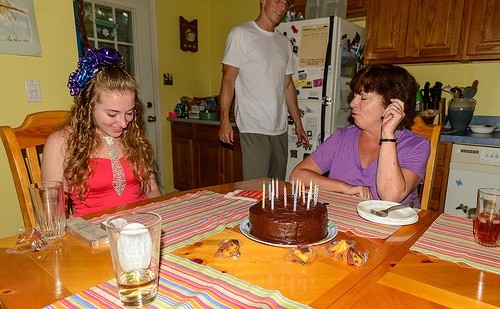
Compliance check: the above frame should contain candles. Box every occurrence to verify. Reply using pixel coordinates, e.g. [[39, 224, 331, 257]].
[[262, 177, 318, 211]]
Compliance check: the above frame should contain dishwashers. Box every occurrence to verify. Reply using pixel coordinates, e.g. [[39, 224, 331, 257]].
[[443, 144, 500, 219]]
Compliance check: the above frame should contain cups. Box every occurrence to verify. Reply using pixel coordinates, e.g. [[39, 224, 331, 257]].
[[28, 181, 67, 240], [104, 212, 162, 305], [420, 111, 437, 125], [474, 188, 500, 247]]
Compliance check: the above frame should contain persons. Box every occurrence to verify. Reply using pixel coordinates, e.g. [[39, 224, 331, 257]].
[[217, 0, 312, 181], [289, 61, 432, 211], [40, 44, 164, 225]]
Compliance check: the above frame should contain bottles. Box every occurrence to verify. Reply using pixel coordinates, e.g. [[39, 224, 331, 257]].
[[183, 98, 189, 119], [448, 97, 477, 131], [414, 85, 422, 115]]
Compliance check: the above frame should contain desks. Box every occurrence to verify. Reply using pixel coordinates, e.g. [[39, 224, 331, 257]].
[[0, 177, 500, 309]]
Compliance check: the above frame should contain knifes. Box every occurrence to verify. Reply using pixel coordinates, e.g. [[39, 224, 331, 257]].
[[422, 81, 444, 111]]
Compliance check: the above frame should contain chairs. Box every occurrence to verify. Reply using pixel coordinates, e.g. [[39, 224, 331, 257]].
[[410, 124, 443, 210], [0, 111, 74, 230]]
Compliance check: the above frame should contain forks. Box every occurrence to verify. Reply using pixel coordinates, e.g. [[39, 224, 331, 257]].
[[370, 199, 418, 217]]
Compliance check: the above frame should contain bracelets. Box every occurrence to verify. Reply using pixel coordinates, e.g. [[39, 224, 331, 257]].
[[378, 138, 399, 147]]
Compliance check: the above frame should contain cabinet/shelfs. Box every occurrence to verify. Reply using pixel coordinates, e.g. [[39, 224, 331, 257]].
[[430, 141, 453, 212], [170, 120, 242, 190], [289, 0, 500, 64]]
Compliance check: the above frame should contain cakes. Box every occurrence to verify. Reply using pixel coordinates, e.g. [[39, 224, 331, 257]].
[[248, 193, 329, 245]]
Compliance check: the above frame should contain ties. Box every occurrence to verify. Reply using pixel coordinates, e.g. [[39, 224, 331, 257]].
[[101, 136, 126, 196]]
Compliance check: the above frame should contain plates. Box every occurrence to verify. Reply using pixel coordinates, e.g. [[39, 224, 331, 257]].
[[238, 216, 339, 248], [356, 199, 418, 225], [469, 124, 498, 134]]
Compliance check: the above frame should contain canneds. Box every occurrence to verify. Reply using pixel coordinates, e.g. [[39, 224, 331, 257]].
[[176, 103, 184, 118]]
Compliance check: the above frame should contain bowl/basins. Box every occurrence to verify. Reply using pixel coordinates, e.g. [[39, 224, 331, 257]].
[[199, 110, 218, 121]]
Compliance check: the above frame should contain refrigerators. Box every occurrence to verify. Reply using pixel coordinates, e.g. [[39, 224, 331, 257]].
[[276, 16, 367, 180]]
